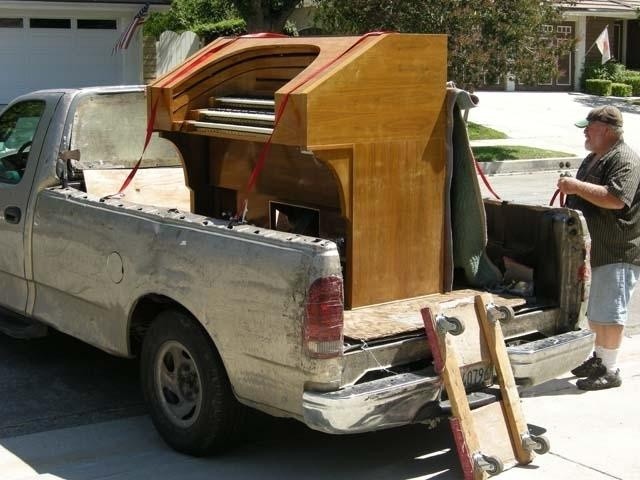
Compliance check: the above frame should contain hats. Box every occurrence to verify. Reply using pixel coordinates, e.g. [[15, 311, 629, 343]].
[[573, 105, 623, 129]]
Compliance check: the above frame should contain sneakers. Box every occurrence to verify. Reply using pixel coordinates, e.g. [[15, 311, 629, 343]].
[[571, 351, 622, 391]]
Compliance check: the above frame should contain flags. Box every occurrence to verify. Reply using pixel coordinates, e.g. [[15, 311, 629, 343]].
[[596, 27, 611, 65]]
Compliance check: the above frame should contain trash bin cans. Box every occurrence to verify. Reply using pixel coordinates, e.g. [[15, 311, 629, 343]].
[[503, 74, 516, 92]]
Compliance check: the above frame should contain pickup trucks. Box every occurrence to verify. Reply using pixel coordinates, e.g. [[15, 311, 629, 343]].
[[0, 83, 599, 456]]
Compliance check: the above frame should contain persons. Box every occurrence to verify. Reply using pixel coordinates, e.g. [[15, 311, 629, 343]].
[[557, 107, 639, 391]]
